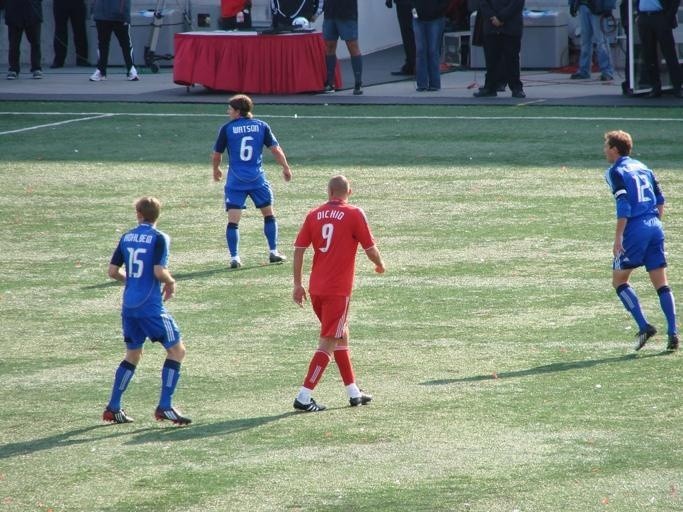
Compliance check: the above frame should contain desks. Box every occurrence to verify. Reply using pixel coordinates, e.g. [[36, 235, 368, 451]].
[[174, 26, 341, 96]]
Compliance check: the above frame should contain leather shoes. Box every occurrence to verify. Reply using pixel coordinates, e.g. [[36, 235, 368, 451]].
[[473, 85, 506, 97], [644, 89, 661, 98], [391, 67, 442, 91], [511, 90, 526, 97]]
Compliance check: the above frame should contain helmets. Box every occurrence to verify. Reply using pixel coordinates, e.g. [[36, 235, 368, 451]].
[[291, 16, 310, 31]]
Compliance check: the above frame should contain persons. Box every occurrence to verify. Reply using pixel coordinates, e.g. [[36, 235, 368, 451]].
[[210, 94, 291, 269], [385, 1, 527, 98], [100, 195, 191, 426], [219, 0, 365, 95], [2, 1, 140, 82], [289, 175, 385, 411], [567, 1, 683, 99], [599, 129, 681, 352]]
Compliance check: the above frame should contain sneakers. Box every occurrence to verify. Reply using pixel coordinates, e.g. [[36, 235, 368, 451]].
[[230, 257, 243, 270], [600, 73, 614, 82], [32, 63, 64, 79], [103, 406, 136, 424], [5, 70, 20, 81], [293, 398, 327, 412], [127, 65, 139, 80], [665, 333, 679, 352], [75, 61, 107, 81], [269, 252, 289, 263], [320, 84, 337, 94], [155, 406, 191, 427], [572, 69, 591, 79], [352, 84, 364, 94], [634, 323, 657, 351], [349, 391, 373, 406]]
